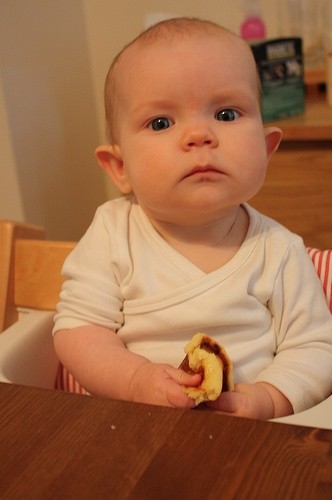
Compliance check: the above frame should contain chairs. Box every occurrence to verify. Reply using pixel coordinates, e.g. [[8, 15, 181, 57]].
[[0, 218, 332, 426]]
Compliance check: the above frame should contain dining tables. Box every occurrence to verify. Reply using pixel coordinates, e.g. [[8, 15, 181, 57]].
[[0, 380, 332, 500]]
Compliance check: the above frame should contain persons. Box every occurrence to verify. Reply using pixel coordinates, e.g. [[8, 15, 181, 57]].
[[52, 18, 331, 429]]
